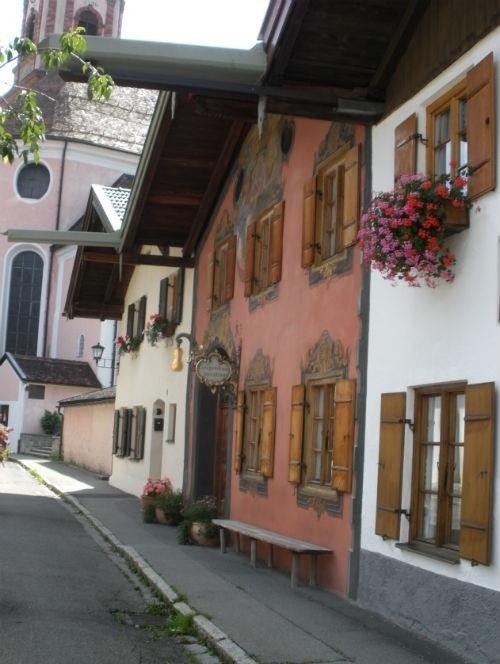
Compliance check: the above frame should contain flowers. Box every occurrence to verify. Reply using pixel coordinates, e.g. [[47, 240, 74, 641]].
[[143, 478, 173, 496], [176, 496, 230, 545], [142, 314, 172, 347], [116, 335, 143, 360], [356, 160, 480, 289]]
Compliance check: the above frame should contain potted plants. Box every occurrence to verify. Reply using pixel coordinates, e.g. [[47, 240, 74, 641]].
[[143, 489, 183, 526]]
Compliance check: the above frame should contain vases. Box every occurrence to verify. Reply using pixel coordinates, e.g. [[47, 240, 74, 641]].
[[141, 495, 154, 511], [162, 323, 174, 336], [187, 522, 220, 545], [442, 205, 469, 240]]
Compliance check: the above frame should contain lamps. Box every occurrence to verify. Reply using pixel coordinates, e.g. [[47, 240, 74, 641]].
[[92, 342, 120, 375], [172, 333, 199, 371]]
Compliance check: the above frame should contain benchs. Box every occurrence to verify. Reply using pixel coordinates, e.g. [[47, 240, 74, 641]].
[[212, 519, 332, 588]]
